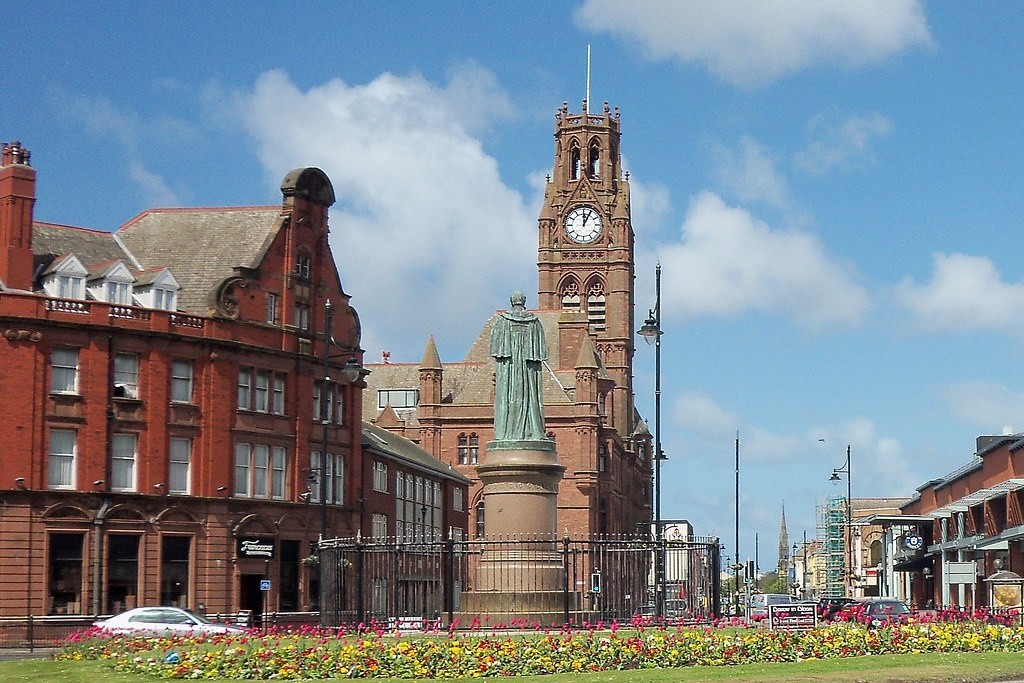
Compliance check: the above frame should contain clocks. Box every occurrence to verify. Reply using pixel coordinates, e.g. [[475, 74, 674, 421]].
[[564, 206, 603, 244]]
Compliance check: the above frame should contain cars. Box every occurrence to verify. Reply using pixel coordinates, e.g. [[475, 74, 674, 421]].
[[633, 582, 880, 625], [857, 599, 913, 632], [93, 606, 245, 641]]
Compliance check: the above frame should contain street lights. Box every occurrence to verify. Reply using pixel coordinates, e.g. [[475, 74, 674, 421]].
[[635, 258, 669, 628], [792, 530, 807, 600], [829, 444, 852, 597]]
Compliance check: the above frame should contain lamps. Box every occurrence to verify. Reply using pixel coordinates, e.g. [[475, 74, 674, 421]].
[[923, 567, 934, 579], [93, 480, 105, 493], [154, 482, 166, 495], [14, 478, 27, 490], [299, 491, 313, 504], [993, 559, 1008, 573], [217, 485, 228, 497]]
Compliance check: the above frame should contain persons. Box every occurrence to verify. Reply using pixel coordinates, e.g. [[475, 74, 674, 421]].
[[489, 290, 548, 441]]
